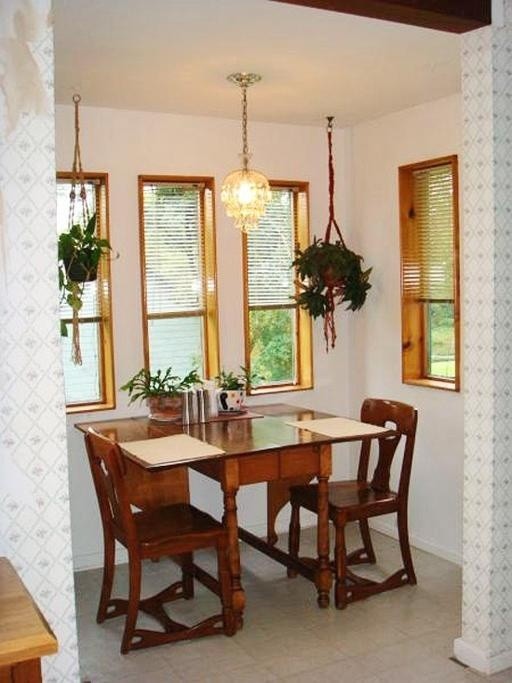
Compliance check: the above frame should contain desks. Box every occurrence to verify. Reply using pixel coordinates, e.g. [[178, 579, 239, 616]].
[[74, 405, 400, 631]]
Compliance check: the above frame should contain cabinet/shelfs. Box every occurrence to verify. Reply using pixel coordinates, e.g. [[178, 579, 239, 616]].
[[1, 556, 58, 683]]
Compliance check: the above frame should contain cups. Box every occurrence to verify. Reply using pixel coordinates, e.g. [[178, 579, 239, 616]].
[[198, 388, 210, 422], [182, 390, 198, 425]]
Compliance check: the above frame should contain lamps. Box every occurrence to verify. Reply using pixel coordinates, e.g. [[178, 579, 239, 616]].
[[221, 72, 270, 231]]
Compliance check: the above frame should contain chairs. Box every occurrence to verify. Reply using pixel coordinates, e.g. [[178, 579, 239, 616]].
[[286, 397, 419, 611], [84, 428, 236, 653]]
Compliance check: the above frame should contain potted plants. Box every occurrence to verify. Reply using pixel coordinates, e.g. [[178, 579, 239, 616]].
[[120, 368, 204, 421], [58, 211, 111, 337], [215, 366, 267, 413], [290, 238, 371, 320]]
